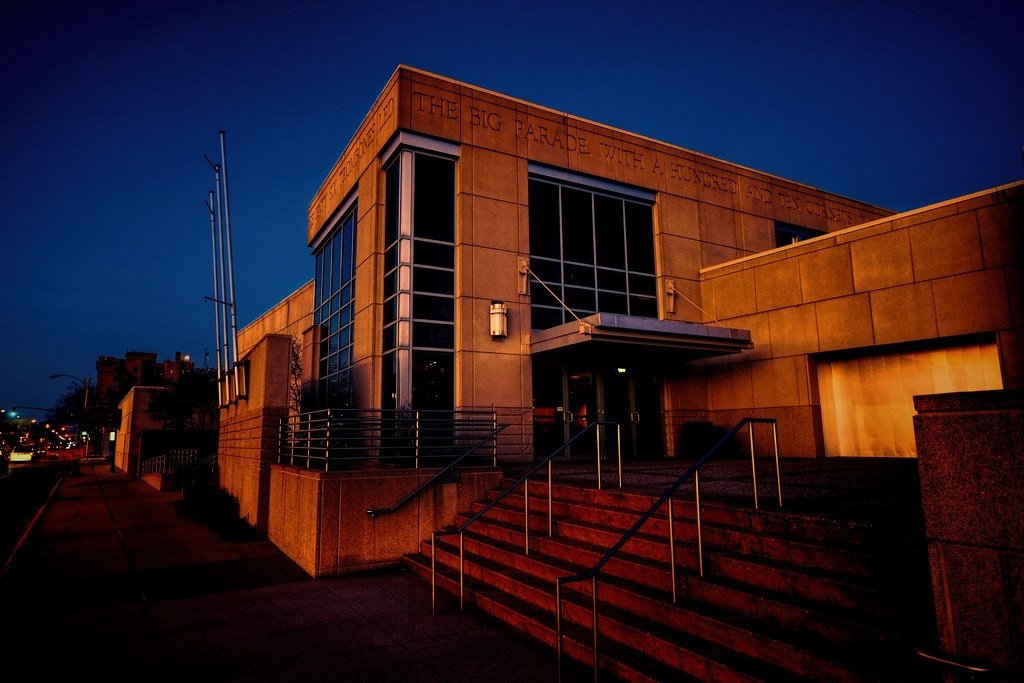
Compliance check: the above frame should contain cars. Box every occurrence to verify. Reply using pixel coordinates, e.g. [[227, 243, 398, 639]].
[[0, 449, 10, 475], [4, 442, 64, 462]]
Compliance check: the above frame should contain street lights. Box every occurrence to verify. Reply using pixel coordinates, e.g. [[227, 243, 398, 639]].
[[49, 373, 90, 458]]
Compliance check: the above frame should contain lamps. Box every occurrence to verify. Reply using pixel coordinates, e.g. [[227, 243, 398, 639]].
[[489, 303, 507, 336]]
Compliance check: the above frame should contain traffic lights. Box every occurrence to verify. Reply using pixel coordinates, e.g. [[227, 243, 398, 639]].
[[11, 407, 16, 417]]
